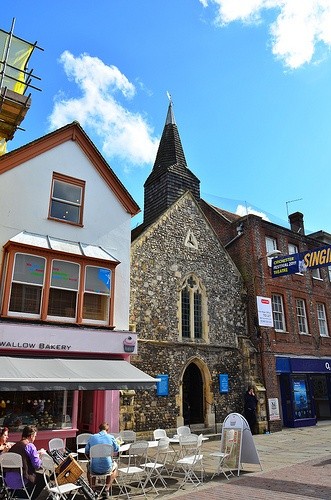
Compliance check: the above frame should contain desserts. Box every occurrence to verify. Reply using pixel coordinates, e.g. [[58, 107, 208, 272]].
[[123, 336, 137, 352], [30, 411, 53, 427]]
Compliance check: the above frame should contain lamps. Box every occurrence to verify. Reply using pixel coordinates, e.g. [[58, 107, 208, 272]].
[[258, 249, 283, 262]]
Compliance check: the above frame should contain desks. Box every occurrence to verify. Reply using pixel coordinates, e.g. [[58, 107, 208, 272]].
[[132, 437, 169, 463], [168, 434, 209, 457]]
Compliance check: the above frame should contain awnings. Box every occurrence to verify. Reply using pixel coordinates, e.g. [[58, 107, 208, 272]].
[[0, 356, 162, 392]]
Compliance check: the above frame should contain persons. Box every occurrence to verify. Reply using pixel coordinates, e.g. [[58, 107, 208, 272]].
[[0, 427, 13, 485], [244, 387, 258, 436], [85, 423, 119, 496], [4, 426, 50, 500]]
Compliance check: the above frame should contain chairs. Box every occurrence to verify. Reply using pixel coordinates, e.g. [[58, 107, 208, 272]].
[[39, 452, 83, 500], [0, 452, 38, 500], [209, 429, 240, 482], [116, 440, 150, 500], [121, 429, 138, 467], [175, 432, 204, 491], [176, 426, 195, 456], [48, 437, 65, 455], [140, 435, 171, 492], [87, 443, 115, 499], [153, 429, 178, 469], [75, 433, 94, 465]]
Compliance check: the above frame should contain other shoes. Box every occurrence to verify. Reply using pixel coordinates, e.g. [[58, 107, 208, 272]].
[[105, 487, 109, 497]]
